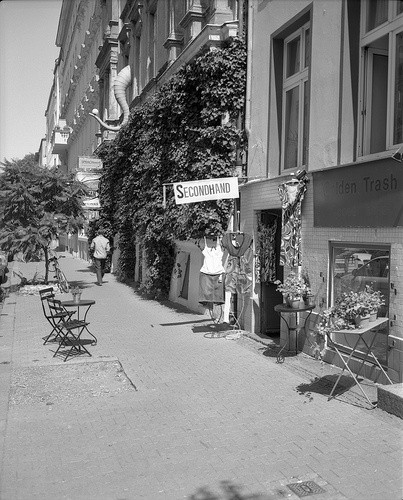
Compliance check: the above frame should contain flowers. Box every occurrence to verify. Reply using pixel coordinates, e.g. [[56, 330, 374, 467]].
[[310, 285, 386, 359], [275, 271, 312, 299]]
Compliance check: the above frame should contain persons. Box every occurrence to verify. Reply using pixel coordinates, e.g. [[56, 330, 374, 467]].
[[91, 229, 111, 285]]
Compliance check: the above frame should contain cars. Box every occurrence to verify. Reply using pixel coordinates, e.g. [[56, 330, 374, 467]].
[[341, 255, 390, 316]]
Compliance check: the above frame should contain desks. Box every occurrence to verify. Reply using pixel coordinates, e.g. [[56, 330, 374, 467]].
[[62, 299, 98, 346], [325, 317, 393, 408], [274, 302, 316, 364]]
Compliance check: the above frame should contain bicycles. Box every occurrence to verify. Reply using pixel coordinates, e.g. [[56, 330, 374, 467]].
[[46, 255, 72, 293]]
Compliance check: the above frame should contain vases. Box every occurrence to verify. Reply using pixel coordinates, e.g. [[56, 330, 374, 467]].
[[356, 309, 379, 329], [287, 296, 300, 309]]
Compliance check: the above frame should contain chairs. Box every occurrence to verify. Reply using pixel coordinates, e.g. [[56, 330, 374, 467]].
[[38, 287, 91, 360]]
